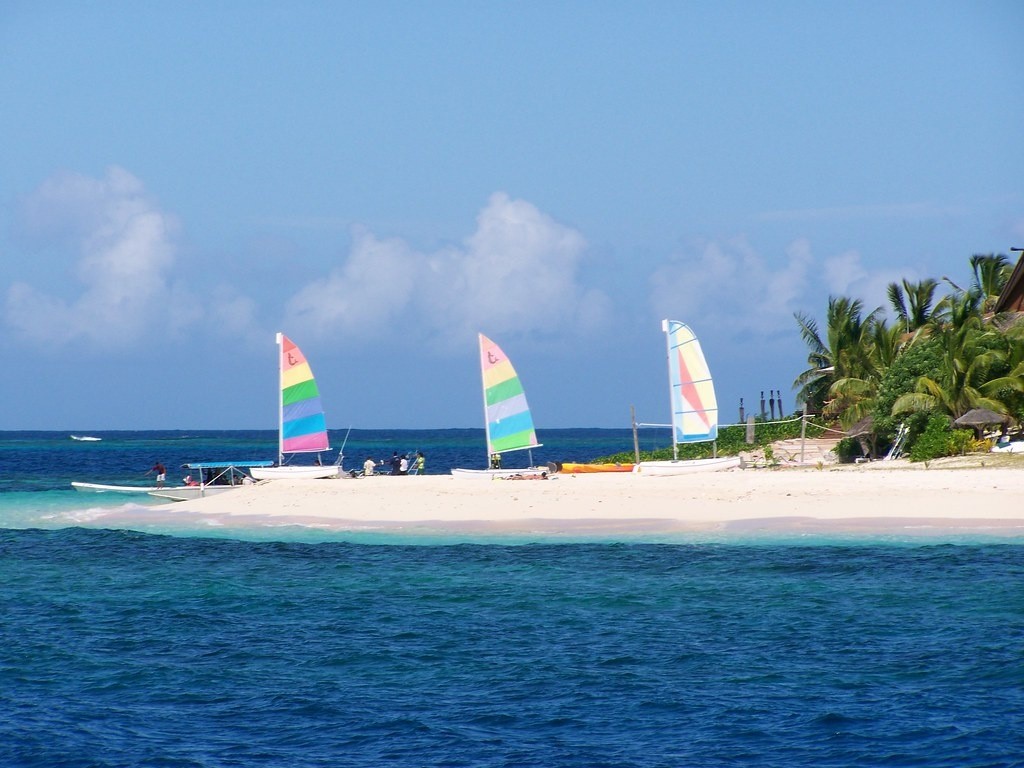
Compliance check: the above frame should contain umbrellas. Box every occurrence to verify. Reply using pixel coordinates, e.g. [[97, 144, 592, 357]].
[[847, 416, 879, 460], [955, 407, 1005, 441]]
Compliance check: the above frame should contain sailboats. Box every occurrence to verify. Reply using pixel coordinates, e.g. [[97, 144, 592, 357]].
[[249, 332, 339, 479], [631, 319, 741, 476], [450, 333, 547, 478]]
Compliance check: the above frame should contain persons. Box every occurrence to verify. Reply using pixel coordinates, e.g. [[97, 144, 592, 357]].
[[412, 452, 425, 475], [204, 468, 245, 485], [144, 461, 166, 489], [315, 460, 320, 466], [387, 451, 408, 475], [363, 456, 382, 476]]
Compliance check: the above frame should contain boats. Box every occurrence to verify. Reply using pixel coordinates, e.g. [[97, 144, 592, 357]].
[[70, 435, 102, 442], [71, 482, 171, 495], [147, 461, 275, 502], [562, 462, 636, 473]]
